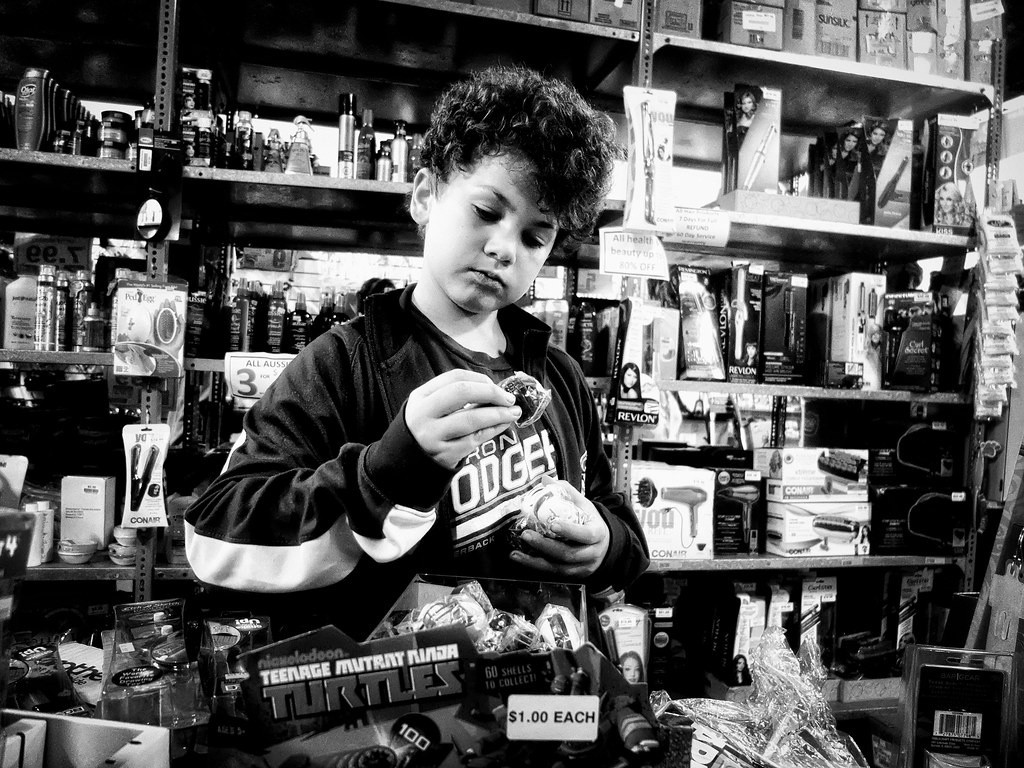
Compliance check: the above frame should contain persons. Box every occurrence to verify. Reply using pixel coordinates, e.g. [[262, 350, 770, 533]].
[[620, 651, 645, 683], [182, 66, 650, 608], [866, 121, 893, 178], [828, 139, 839, 180], [839, 129, 864, 187], [736, 92, 757, 150], [729, 654, 753, 686], [619, 362, 644, 400], [148, 484, 160, 497], [934, 182, 971, 227]]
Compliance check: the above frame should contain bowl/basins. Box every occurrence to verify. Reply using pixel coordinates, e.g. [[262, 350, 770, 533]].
[[59, 539, 99, 553], [57, 550, 94, 564]]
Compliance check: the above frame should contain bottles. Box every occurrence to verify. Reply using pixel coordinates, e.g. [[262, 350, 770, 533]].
[[1, 67, 427, 183], [0, 261, 358, 359], [35, 500, 54, 563], [23, 503, 42, 568]]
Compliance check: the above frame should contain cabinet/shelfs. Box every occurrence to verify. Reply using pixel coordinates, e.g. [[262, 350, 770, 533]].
[[3, 1, 1024, 768]]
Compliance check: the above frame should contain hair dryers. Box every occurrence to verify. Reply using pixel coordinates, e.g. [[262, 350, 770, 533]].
[[625, 454, 769, 560]]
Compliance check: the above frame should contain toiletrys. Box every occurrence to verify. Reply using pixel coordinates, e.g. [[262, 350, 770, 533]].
[[2, 51, 607, 383]]
